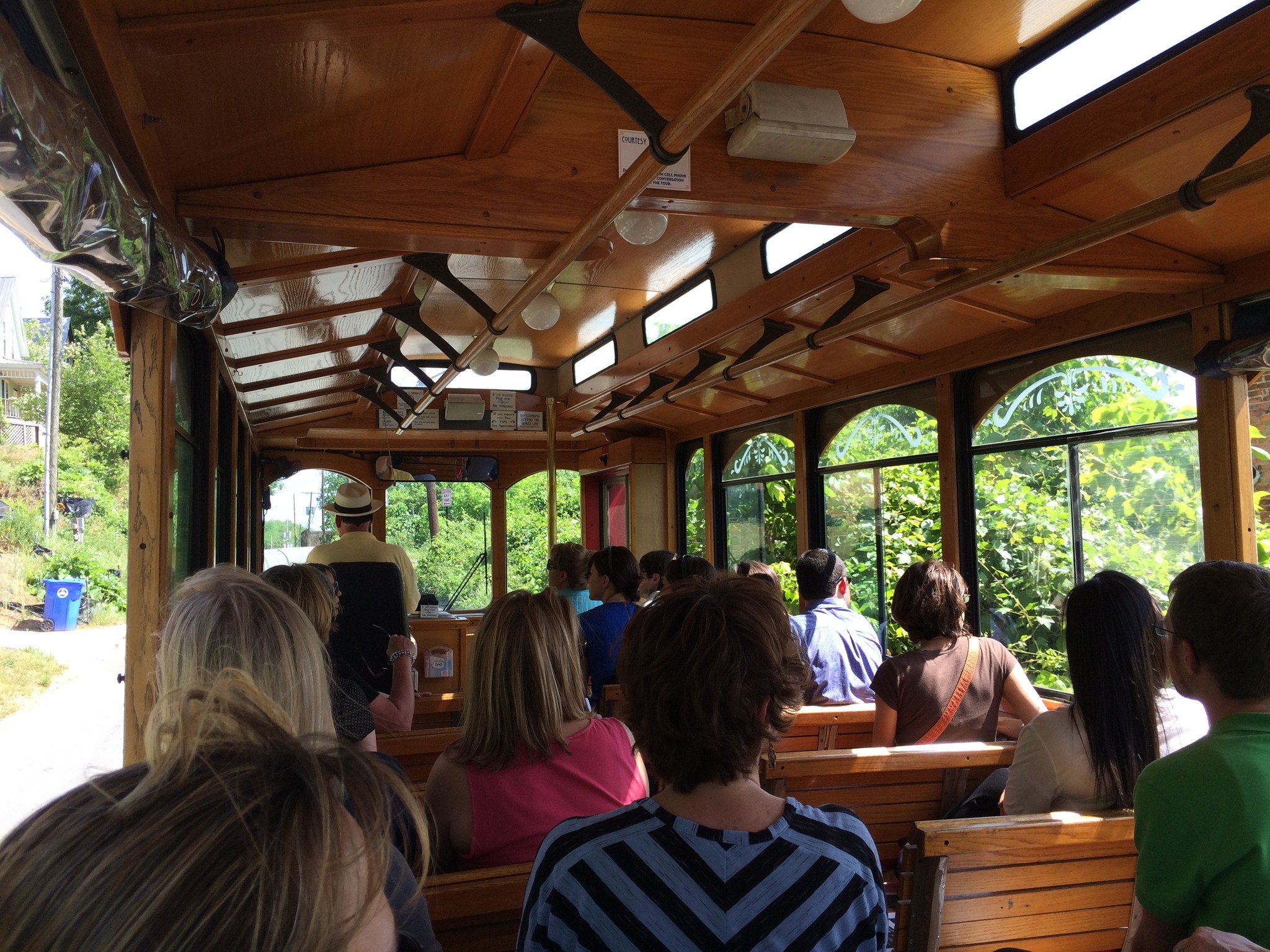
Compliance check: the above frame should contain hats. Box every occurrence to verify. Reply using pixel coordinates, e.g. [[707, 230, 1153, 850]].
[[320, 484, 387, 518]]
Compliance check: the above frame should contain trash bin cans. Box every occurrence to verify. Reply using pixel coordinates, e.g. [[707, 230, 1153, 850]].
[[40, 578, 86, 632]]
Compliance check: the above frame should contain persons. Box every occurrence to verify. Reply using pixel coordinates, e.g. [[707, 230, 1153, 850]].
[[868, 557, 1049, 746], [882, 570, 1210, 895], [146, 565, 450, 951], [425, 587, 648, 869], [783, 548, 893, 704], [544, 542, 792, 711], [304, 483, 421, 660], [301, 563, 433, 734], [0, 670, 431, 951], [514, 570, 890, 952], [255, 565, 377, 752], [376, 456, 498, 481], [1120, 557, 1270, 952]]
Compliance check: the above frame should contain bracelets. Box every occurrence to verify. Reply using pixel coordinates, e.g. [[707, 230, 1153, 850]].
[[390, 649, 416, 666]]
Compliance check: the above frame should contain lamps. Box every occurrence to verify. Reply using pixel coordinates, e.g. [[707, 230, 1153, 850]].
[[520, 281, 561, 331], [613, 210, 668, 245], [470, 335, 500, 377]]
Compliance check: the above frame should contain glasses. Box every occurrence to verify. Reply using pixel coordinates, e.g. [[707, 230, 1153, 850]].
[[1146, 620, 1204, 670], [546, 558, 564, 571], [680, 555, 705, 569]]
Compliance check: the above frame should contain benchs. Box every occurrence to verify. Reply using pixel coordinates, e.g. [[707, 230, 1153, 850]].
[[891, 803, 1139, 952], [412, 862, 537, 952], [757, 739, 1018, 910], [770, 700, 877, 755], [376, 726, 468, 800]]
